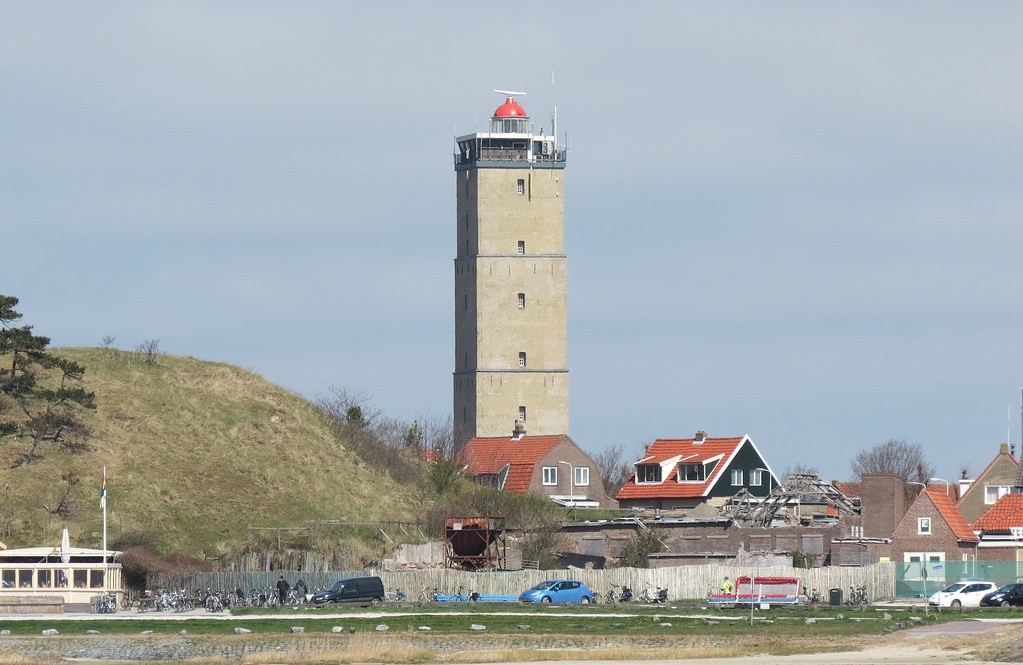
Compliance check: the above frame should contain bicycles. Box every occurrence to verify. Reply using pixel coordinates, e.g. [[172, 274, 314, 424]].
[[97, 584, 306, 615], [383, 585, 407, 602], [797, 584, 827, 605], [590, 582, 672, 605], [415, 586, 446, 603], [448, 585, 482, 601], [845, 581, 870, 605]]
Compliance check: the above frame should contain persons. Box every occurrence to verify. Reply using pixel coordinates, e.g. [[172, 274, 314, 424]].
[[10, 581, 14, 587], [22, 581, 30, 587], [293, 580, 308, 604], [396, 589, 405, 602], [720, 577, 733, 595], [277, 576, 290, 605]]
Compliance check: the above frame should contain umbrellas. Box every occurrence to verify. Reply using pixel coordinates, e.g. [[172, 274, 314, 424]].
[[60, 528, 70, 585]]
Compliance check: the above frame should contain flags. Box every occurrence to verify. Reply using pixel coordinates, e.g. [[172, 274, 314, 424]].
[[100, 477, 106, 511]]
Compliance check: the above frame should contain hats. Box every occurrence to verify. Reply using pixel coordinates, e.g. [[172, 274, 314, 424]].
[[279, 576, 283, 579]]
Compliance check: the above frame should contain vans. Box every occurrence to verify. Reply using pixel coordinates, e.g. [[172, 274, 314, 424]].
[[310, 575, 386, 610]]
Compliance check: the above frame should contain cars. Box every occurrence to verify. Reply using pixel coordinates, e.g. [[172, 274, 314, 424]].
[[980, 581, 1023, 608], [928, 580, 999, 608], [518, 579, 593, 605]]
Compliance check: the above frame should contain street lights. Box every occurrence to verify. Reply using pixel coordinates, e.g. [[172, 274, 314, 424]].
[[929, 477, 949, 495], [755, 467, 771, 495], [557, 460, 573, 503]]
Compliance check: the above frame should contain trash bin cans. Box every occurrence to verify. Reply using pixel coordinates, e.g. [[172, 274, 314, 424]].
[[829, 588, 844, 606]]
